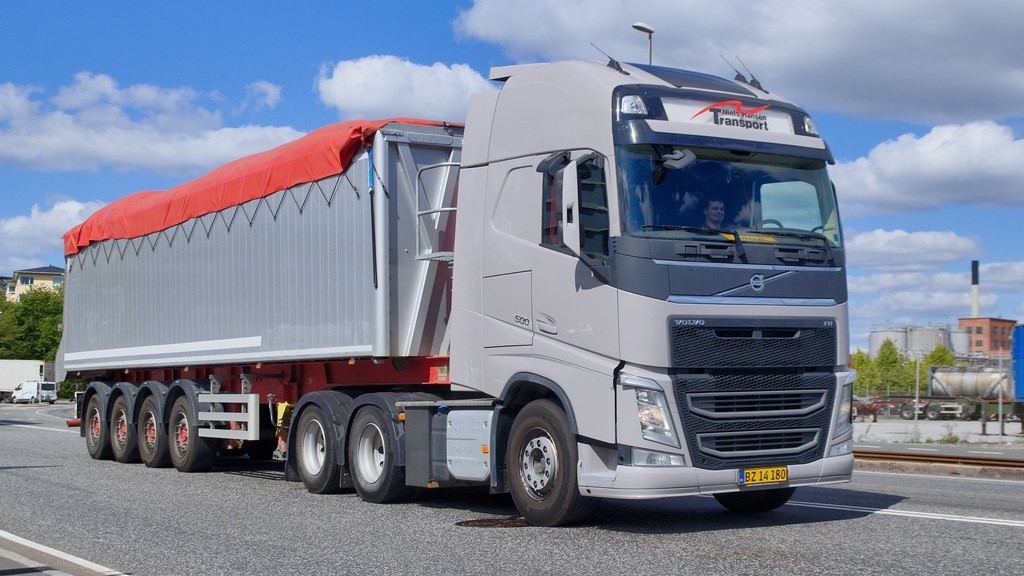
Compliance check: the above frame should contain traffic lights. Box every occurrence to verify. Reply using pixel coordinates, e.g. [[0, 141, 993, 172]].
[[39, 365, 44, 376]]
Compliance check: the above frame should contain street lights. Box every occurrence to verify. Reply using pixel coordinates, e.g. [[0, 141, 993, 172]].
[[632, 20, 657, 65]]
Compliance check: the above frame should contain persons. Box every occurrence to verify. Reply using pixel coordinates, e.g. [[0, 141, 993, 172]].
[[692, 197, 728, 228]]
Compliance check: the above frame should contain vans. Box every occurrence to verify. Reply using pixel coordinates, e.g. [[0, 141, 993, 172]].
[[11, 380, 57, 405]]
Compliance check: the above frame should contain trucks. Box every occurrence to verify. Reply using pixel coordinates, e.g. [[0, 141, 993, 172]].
[[0, 358, 45, 403]]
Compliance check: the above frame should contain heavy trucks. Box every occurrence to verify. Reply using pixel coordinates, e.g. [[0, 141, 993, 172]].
[[54, 58, 861, 527], [851, 322, 1024, 434]]
